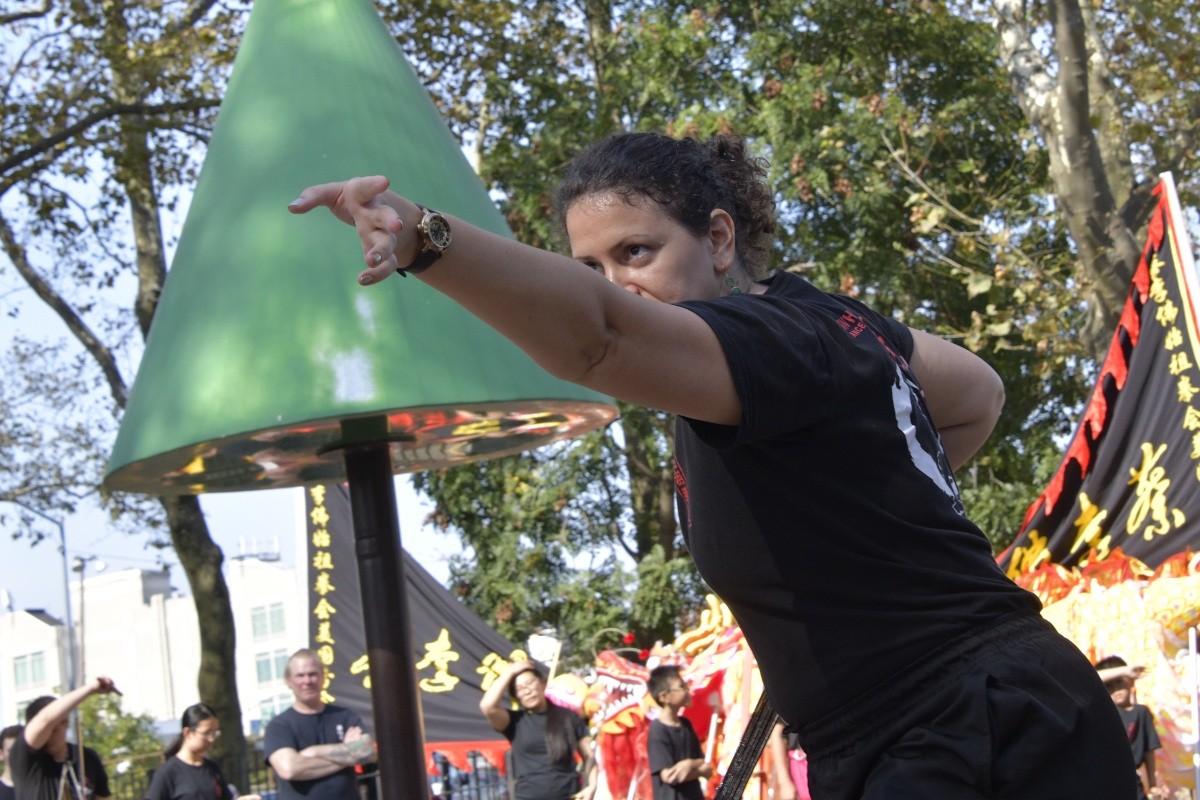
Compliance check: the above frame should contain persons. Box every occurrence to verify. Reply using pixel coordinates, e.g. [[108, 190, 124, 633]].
[[264, 648, 379, 799], [9, 674, 114, 800], [760, 723, 811, 800], [479, 659, 599, 800], [0, 724, 26, 800], [1092, 654, 1163, 800], [287, 131, 1152, 800], [147, 702, 238, 800], [647, 663, 714, 800]]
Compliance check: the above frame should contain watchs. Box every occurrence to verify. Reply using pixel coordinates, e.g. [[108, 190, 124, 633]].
[[396, 202, 454, 278]]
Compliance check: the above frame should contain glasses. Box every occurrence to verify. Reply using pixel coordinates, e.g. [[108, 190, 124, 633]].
[[191, 729, 221, 739]]
